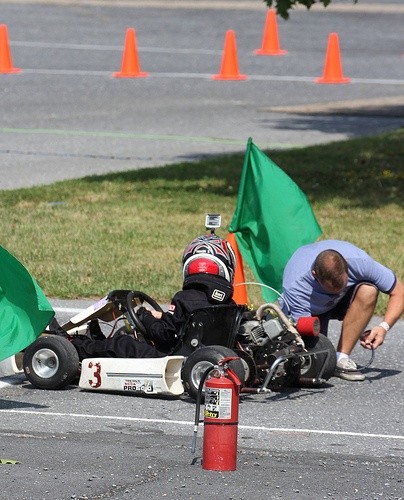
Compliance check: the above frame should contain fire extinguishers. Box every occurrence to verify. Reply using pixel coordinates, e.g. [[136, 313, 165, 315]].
[[190, 356, 242, 472]]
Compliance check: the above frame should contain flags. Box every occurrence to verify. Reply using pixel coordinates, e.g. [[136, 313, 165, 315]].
[[227, 141, 323, 304], [0, 245, 55, 362]]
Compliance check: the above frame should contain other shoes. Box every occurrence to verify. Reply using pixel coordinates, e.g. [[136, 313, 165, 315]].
[[49, 319, 69, 342], [86, 319, 107, 340]]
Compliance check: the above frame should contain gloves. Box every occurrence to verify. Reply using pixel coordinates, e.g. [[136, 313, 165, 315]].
[[136, 307, 153, 324]]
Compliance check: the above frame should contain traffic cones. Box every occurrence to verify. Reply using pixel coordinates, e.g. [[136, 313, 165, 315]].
[[212, 29, 248, 80], [314, 33, 351, 84], [253, 9, 288, 56], [0, 23, 21, 74], [113, 27, 148, 78]]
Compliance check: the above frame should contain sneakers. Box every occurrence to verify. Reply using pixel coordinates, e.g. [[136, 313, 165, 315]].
[[334, 358, 365, 381]]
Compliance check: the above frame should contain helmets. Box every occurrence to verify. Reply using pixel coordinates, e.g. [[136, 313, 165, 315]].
[[181, 234, 235, 284]]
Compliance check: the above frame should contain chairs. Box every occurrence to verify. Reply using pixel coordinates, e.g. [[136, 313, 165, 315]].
[[171, 304, 248, 356]]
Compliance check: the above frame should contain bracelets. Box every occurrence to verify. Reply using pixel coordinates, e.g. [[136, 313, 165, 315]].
[[379, 322, 391, 332]]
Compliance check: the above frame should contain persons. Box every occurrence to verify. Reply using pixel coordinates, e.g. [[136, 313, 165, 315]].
[[49, 235, 241, 362], [277, 239, 404, 380]]
